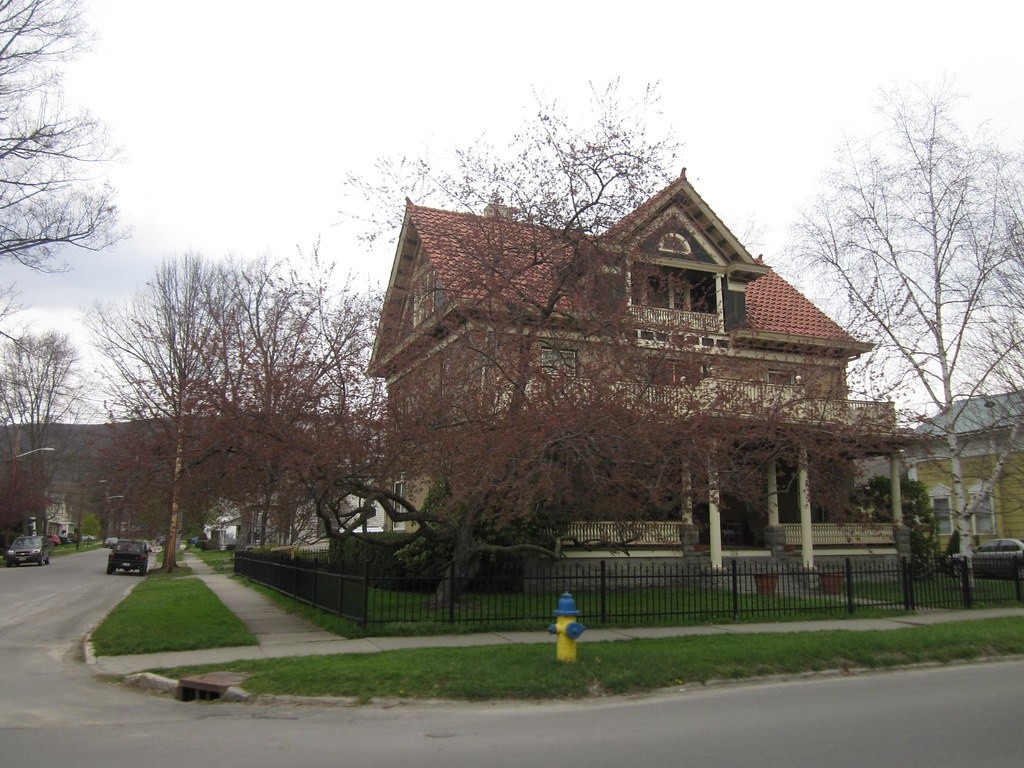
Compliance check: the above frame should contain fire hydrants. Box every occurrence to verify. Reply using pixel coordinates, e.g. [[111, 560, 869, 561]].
[[547, 591, 584, 665]]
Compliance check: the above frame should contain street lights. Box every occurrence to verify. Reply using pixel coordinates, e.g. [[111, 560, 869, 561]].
[[103, 496, 122, 546], [77, 480, 106, 549], [4, 448, 55, 560]]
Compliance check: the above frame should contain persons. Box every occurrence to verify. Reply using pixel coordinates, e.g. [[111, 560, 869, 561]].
[[191, 537, 198, 544]]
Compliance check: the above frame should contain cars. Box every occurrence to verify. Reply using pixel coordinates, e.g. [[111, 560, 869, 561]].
[[82, 534, 95, 541], [105, 537, 118, 549], [6, 536, 51, 566], [45, 534, 61, 546], [107, 539, 153, 575], [156, 537, 167, 545], [944, 538, 1024, 581]]
